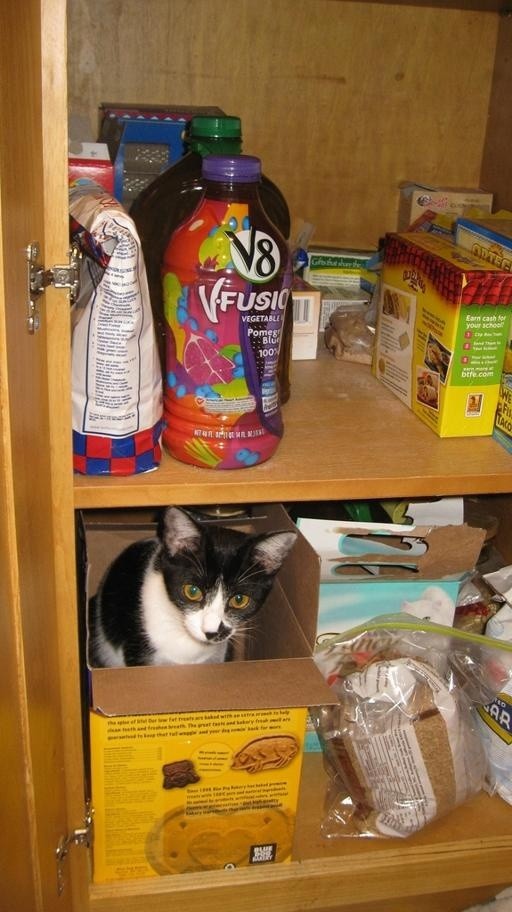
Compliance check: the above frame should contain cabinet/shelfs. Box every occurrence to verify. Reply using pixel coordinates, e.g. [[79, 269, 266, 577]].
[[61, 341, 510, 911]]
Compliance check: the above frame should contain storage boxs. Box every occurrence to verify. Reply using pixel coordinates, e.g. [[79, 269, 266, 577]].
[[292, 510, 486, 757], [74, 500, 342, 891]]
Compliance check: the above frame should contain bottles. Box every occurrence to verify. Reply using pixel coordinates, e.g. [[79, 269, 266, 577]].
[[124, 112, 296, 404], [155, 152, 295, 470]]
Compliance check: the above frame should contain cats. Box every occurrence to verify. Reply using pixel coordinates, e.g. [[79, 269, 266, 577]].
[[87, 503, 300, 668]]
